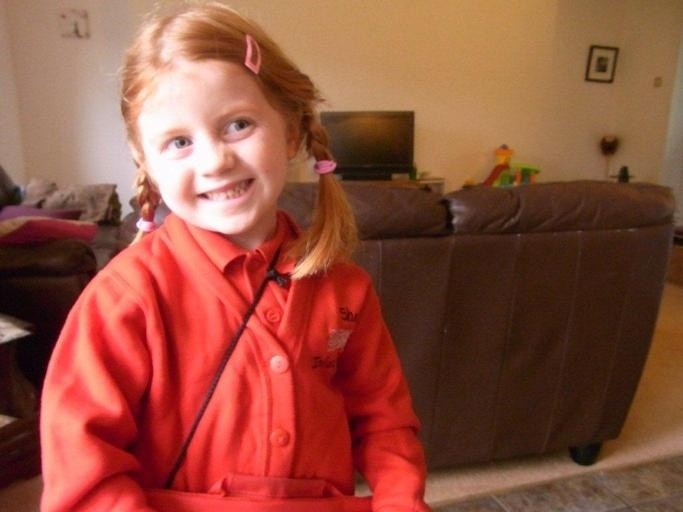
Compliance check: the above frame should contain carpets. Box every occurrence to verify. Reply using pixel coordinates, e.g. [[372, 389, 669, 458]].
[[0, 274, 681, 512]]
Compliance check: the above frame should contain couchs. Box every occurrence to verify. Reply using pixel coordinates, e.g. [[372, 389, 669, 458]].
[[0, 165, 121, 382], [275, 178, 681, 467]]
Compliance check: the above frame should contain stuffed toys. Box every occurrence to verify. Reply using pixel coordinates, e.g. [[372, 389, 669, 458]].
[[480, 143, 540, 189]]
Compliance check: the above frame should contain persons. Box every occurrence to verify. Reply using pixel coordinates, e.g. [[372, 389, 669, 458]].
[[36, 4, 432, 512]]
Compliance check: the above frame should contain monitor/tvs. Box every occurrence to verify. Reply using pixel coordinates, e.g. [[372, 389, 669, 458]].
[[320, 110, 413, 185]]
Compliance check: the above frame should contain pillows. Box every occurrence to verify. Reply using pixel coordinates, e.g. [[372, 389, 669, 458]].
[[0, 176, 118, 243]]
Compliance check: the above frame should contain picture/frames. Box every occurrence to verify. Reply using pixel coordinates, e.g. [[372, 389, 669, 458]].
[[586, 44, 620, 84]]
